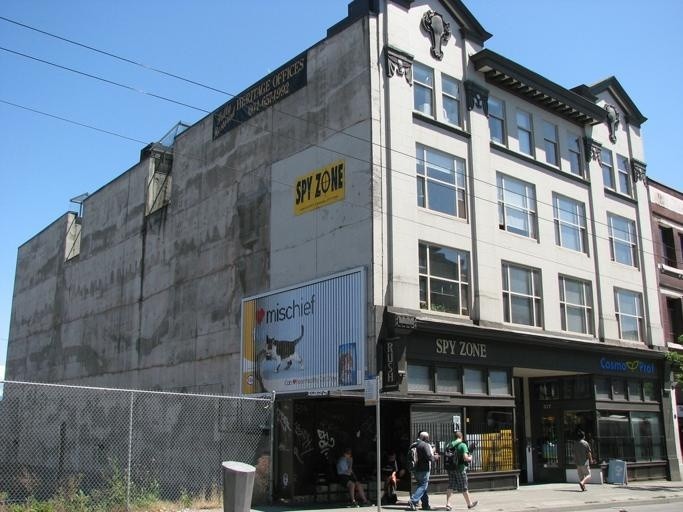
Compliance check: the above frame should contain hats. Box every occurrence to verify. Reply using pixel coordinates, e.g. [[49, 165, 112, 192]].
[[344, 447, 352, 454]]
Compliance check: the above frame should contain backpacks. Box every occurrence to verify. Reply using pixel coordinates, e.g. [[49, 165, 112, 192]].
[[444, 440, 462, 470], [407, 441, 425, 471]]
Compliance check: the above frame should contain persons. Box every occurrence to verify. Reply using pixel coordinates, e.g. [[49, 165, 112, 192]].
[[574, 429, 593, 491], [444, 432, 479, 511], [408, 431, 439, 511], [336, 448, 375, 508], [383, 450, 399, 501]]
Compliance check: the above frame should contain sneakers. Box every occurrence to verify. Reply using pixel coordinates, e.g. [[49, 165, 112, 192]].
[[468, 501, 478, 508], [351, 502, 360, 508], [579, 482, 585, 491], [446, 505, 452, 511], [363, 501, 375, 506], [408, 498, 418, 511], [422, 504, 431, 509]]
